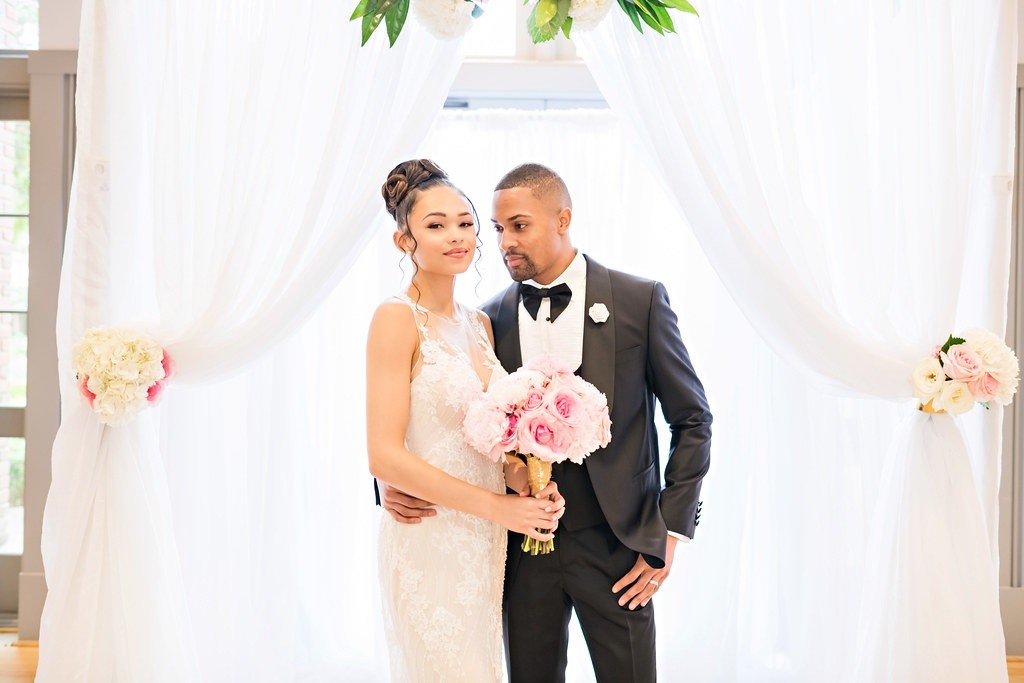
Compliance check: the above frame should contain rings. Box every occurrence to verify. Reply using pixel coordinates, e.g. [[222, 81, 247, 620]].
[[650, 580, 658, 585]]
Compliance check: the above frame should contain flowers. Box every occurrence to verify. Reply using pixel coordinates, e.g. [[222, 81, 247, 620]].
[[589, 303, 610, 324], [70, 320, 174, 426], [910, 329, 1021, 415], [349, 0, 701, 49], [458, 361, 613, 556]]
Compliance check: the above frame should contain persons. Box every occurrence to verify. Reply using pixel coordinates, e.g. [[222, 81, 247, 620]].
[[365, 160, 566, 683], [384, 164, 714, 683]]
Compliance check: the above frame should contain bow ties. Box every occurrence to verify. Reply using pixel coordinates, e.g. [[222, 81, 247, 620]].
[[520, 282, 572, 323]]
